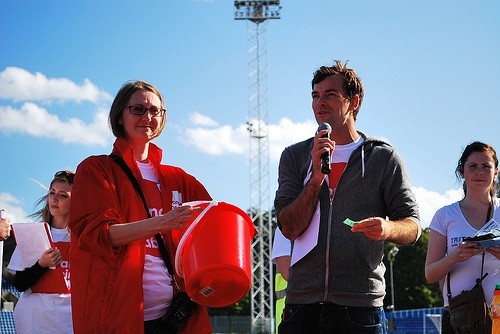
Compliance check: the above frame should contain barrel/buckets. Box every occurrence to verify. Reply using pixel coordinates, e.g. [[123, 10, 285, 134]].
[[171, 200, 255, 308]]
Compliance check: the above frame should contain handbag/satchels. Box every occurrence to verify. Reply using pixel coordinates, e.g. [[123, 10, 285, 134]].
[[162, 291, 197, 331], [449, 278, 492, 334]]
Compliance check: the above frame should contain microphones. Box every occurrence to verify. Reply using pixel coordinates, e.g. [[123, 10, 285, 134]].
[[317, 122, 332, 174]]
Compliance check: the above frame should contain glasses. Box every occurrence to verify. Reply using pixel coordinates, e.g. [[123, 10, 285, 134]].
[[55, 171, 76, 182], [124, 104, 166, 117]]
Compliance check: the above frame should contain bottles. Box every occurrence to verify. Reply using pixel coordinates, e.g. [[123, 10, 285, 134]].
[[489, 284, 500, 334]]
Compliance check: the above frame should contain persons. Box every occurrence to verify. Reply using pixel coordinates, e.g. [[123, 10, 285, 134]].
[[270, 226, 293, 334], [0, 83, 222, 334], [423, 143, 500, 333], [276, 61, 421, 334]]
